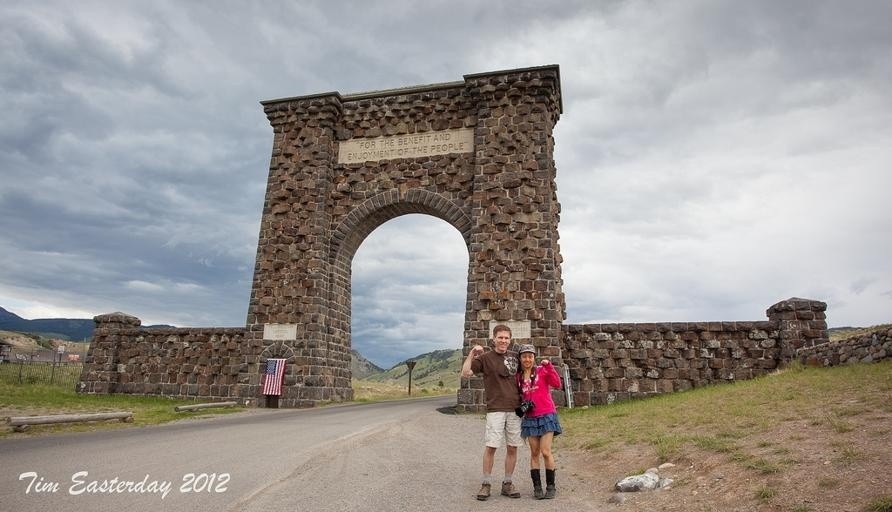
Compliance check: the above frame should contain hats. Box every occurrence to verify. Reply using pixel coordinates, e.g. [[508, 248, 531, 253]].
[[518, 344, 536, 355]]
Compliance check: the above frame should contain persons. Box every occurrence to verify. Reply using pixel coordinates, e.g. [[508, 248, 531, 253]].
[[516, 343, 564, 500], [461, 324, 529, 500]]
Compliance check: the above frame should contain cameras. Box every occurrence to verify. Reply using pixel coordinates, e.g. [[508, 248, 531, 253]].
[[515, 399, 535, 418]]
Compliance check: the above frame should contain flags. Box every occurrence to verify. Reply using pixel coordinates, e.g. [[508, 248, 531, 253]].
[[262, 358, 286, 396]]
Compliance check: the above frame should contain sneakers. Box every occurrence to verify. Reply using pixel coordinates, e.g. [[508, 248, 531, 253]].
[[533, 485, 544, 499], [477, 483, 490, 501], [501, 480, 520, 498], [544, 484, 556, 498]]
